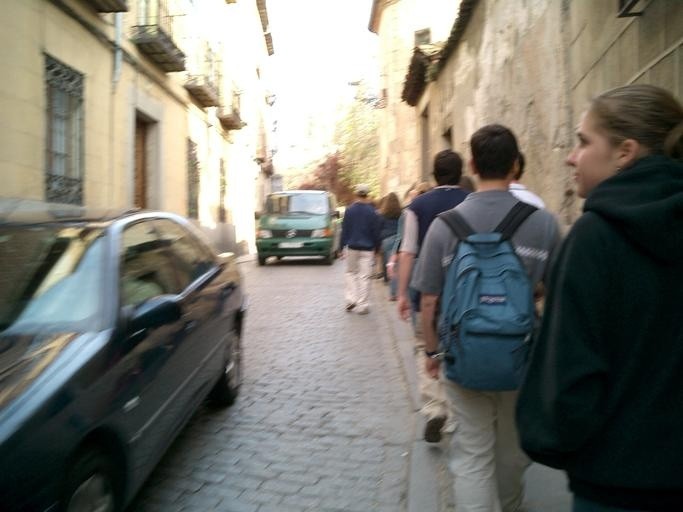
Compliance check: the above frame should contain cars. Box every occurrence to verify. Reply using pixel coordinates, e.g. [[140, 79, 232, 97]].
[[0, 207, 246, 512]]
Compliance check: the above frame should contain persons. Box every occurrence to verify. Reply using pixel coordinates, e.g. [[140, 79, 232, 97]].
[[511, 83, 683, 511], [407, 123, 564, 512], [328, 150, 548, 315], [394, 148, 472, 444]]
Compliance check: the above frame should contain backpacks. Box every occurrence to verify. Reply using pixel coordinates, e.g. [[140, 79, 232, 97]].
[[433, 201, 540, 390]]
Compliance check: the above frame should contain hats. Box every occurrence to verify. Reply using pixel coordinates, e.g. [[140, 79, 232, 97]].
[[355, 183, 369, 195]]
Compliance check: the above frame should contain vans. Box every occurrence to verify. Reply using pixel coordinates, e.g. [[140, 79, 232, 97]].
[[255, 190, 340, 267]]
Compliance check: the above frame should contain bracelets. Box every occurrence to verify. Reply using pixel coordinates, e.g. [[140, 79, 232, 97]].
[[426, 349, 442, 361]]
